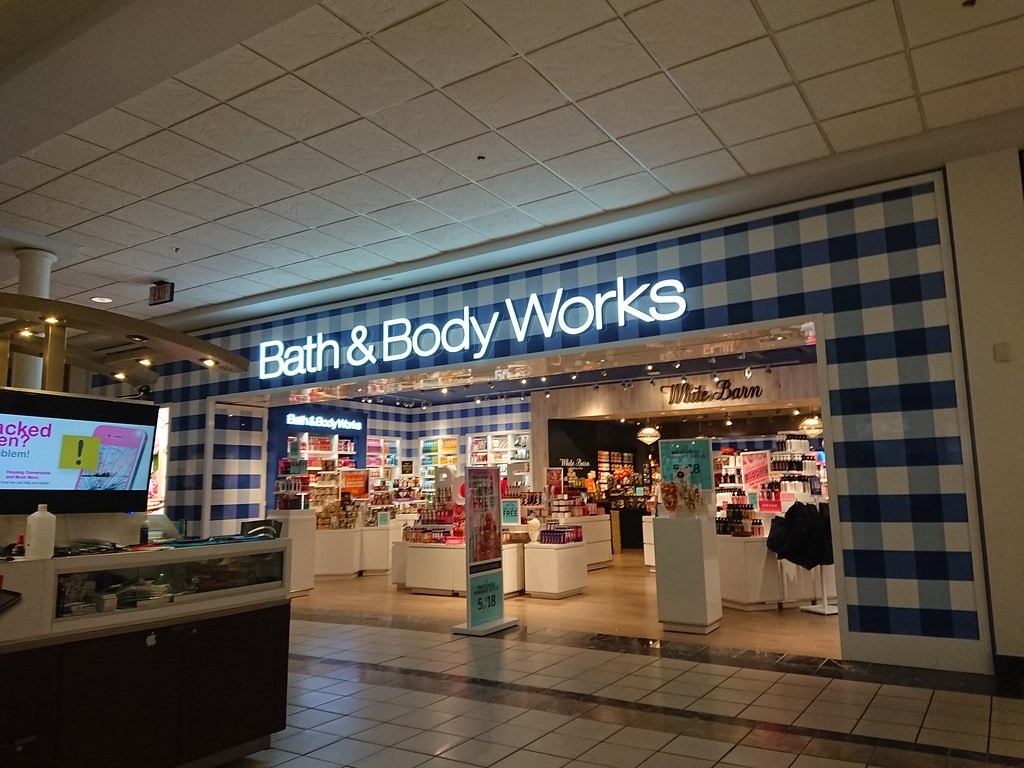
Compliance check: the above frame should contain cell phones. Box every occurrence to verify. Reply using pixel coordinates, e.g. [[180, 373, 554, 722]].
[[74, 425, 148, 490]]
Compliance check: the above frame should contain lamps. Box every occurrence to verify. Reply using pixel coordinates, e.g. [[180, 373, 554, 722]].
[[636, 416, 660, 446], [797, 406, 823, 438]]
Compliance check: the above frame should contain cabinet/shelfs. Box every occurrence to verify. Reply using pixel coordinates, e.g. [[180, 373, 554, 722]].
[[264, 430, 835, 634]]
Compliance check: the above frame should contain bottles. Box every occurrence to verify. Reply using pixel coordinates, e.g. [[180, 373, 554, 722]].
[[277, 437, 597, 543], [11, 535, 25, 556], [714, 434, 826, 537], [140, 514, 149, 545], [24, 504, 56, 559]]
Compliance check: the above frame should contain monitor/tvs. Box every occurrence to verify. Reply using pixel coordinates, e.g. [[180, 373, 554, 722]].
[[0, 389, 160, 516]]
